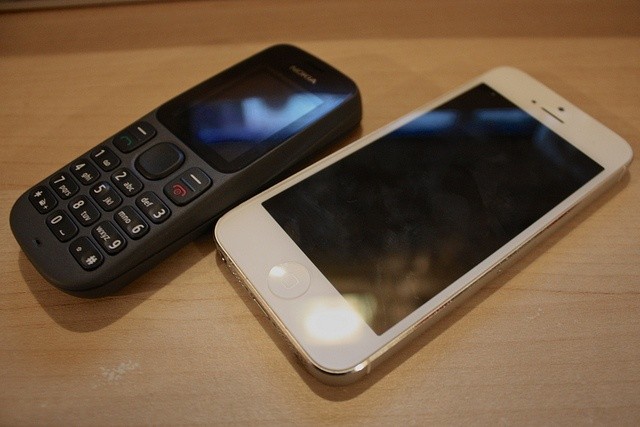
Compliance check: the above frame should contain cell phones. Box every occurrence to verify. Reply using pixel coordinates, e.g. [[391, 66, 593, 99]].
[[213, 64, 634, 386], [9, 43, 363, 299]]
